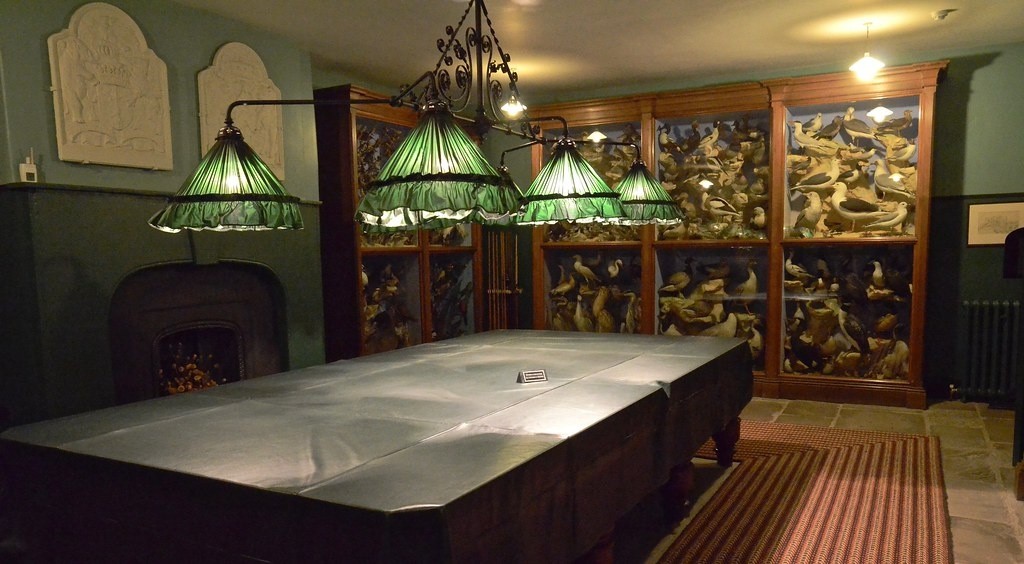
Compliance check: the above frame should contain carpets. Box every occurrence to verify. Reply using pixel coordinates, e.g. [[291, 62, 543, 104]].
[[656, 418, 956, 564]]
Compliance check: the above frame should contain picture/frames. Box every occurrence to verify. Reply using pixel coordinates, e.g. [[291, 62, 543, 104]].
[[966, 201, 1024, 249]]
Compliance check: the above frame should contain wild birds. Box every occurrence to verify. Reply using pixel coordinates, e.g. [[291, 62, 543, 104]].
[[656, 257, 766, 374], [550, 254, 642, 334], [786, 107, 917, 238], [657, 118, 771, 239], [783, 252, 910, 380]]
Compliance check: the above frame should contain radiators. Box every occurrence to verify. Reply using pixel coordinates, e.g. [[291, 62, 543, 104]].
[[949, 299, 1024, 404]]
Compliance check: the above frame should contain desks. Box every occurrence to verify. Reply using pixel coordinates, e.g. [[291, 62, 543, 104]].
[[0, 329, 756, 564]]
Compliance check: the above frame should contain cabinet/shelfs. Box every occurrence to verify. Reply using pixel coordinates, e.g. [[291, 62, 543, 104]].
[[524, 58, 952, 411], [312, 87, 487, 363]]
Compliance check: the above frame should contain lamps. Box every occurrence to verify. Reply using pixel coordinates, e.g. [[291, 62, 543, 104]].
[[499, 90, 527, 116], [848, 21, 884, 83], [147, 1, 684, 235]]
